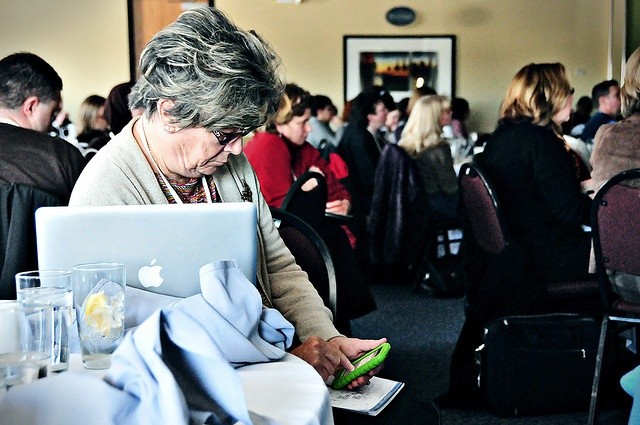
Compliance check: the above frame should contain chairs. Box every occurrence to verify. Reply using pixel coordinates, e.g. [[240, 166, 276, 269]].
[[0, 187, 67, 301], [283, 172, 326, 235], [456, 161, 609, 413], [373, 138, 476, 294], [583, 167, 640, 424], [261, 207, 339, 324]]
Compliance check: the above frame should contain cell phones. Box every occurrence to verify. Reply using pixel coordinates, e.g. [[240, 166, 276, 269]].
[[334, 343, 391, 388]]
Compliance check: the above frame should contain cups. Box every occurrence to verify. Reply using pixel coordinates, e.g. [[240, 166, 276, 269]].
[[73, 263, 126, 369], [0, 300, 53, 390], [14, 270, 72, 377]]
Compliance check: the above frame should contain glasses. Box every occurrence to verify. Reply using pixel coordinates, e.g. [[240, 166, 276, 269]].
[[211, 125, 258, 145], [290, 92, 310, 106]]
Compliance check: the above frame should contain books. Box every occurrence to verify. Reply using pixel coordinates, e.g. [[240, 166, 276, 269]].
[[327, 376, 406, 417]]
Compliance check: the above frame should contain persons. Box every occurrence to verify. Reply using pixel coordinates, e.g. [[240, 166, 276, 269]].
[[338, 89, 387, 219], [242, 82, 356, 257], [580, 77, 623, 142], [449, 96, 470, 138], [0, 50, 86, 299], [588, 46, 639, 196], [379, 99, 404, 145], [46, 102, 69, 142], [103, 81, 136, 136], [68, 6, 388, 391], [473, 59, 628, 367], [399, 94, 466, 227], [307, 94, 348, 150], [562, 96, 592, 135], [75, 93, 112, 160]]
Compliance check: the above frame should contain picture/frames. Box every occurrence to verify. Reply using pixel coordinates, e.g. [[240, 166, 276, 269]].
[[341, 33, 457, 107]]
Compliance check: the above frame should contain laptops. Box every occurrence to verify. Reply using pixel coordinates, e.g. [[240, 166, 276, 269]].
[[35, 202, 256, 298]]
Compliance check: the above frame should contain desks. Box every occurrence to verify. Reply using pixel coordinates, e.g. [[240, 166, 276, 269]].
[[0, 287, 330, 425]]
